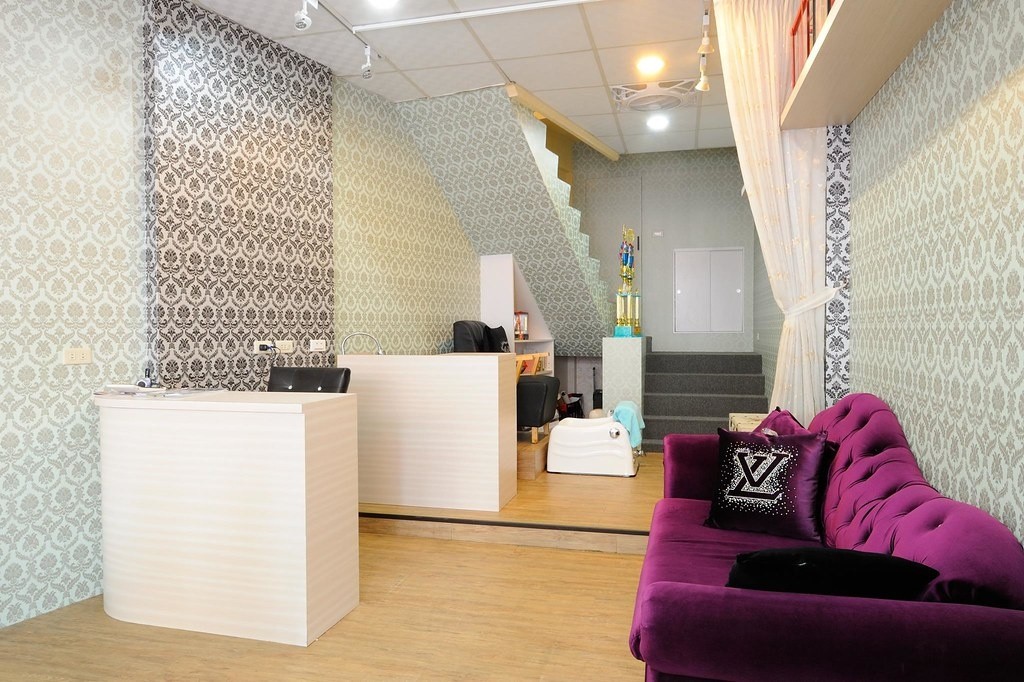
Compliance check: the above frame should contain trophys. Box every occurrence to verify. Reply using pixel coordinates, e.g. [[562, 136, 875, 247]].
[[614, 224, 642, 337]]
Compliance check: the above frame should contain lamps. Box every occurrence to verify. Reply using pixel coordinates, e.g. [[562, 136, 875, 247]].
[[612, 10, 714, 122]]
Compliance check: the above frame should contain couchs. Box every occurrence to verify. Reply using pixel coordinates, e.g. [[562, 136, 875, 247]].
[[627, 389, 1024, 682], [454, 320, 561, 444]]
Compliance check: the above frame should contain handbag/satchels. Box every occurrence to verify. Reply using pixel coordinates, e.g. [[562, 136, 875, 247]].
[[558, 392, 585, 420]]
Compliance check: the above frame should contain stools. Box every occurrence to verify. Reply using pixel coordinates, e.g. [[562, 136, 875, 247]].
[[547, 417, 638, 477]]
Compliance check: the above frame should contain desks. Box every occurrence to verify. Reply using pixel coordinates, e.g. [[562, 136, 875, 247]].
[[727, 411, 769, 437]]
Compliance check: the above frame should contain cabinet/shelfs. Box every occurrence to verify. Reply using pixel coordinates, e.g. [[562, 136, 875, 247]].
[[513, 337, 555, 378]]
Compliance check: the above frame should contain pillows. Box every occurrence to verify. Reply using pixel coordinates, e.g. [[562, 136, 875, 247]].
[[701, 425, 830, 546], [722, 540, 937, 602], [483, 324, 511, 351]]
